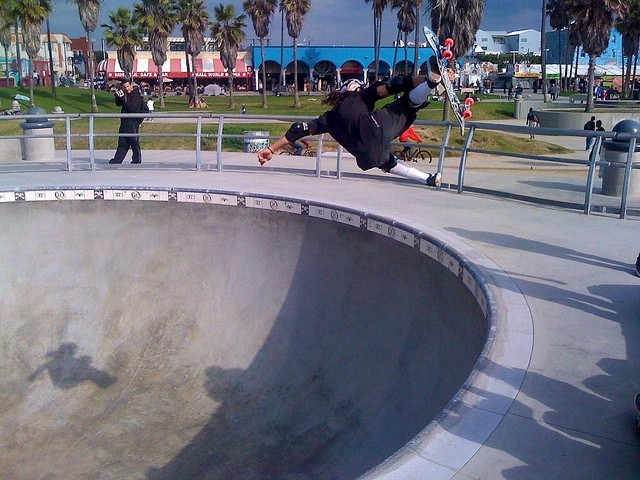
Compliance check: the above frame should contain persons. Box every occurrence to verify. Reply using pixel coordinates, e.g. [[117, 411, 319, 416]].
[[606, 85, 614, 95], [477, 81, 480, 87], [256, 73, 440, 188], [556, 83, 560, 99], [59, 74, 66, 87], [31, 68, 37, 85], [601, 90, 607, 101], [594, 84, 599, 98], [466, 78, 469, 86], [579, 83, 586, 103], [525, 106, 540, 140], [189, 97, 195, 106], [108, 79, 149, 165], [240, 103, 247, 114], [200, 84, 204, 93], [507, 84, 513, 99], [154, 85, 159, 92], [550, 83, 556, 101], [197, 85, 200, 93], [147, 97, 156, 121], [199, 97, 207, 108], [613, 86, 621, 95], [398, 123, 423, 163], [514, 83, 524, 96], [259, 82, 263, 94], [70, 72, 75, 86], [589, 120, 607, 167], [489, 82, 495, 93], [583, 114, 594, 148], [168, 83, 172, 90], [597, 85, 602, 98]]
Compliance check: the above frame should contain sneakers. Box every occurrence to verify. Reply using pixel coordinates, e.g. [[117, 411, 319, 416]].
[[426, 172, 441, 187], [427, 56, 442, 89]]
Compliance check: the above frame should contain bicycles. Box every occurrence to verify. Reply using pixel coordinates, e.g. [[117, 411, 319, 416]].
[[279, 144, 317, 157], [392, 141, 432, 164]]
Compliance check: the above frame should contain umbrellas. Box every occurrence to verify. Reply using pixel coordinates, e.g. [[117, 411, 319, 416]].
[[157, 77, 173, 82]]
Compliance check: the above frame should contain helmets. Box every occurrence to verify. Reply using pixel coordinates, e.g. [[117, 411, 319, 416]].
[[340, 78, 366, 94]]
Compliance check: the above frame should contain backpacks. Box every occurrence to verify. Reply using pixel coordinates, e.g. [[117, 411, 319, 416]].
[[135, 95, 149, 124]]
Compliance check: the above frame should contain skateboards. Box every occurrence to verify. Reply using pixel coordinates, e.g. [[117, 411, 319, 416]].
[[422, 25, 474, 136]]
